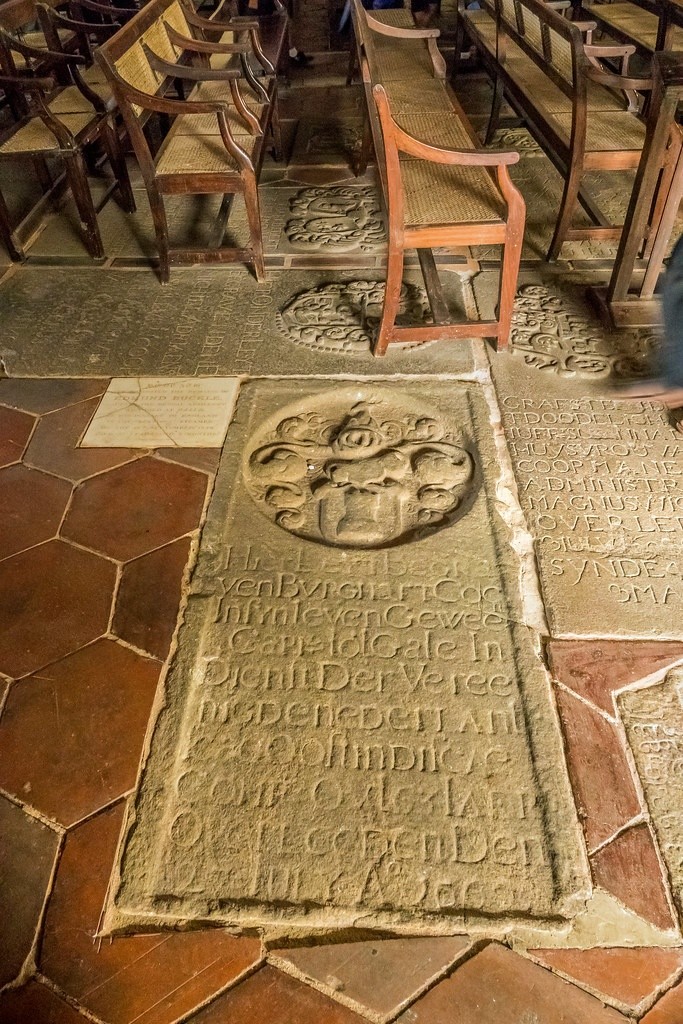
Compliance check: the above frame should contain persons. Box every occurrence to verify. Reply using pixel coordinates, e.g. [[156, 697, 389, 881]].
[[248, 0, 313, 69]]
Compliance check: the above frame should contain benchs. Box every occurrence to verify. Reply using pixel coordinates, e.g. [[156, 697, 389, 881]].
[[93, 0, 281, 285], [355, 0, 526, 358], [577, 0, 683, 92], [455, 0, 597, 146], [182, 0, 299, 164], [0, 0, 184, 261], [484, 0, 681, 260]]
